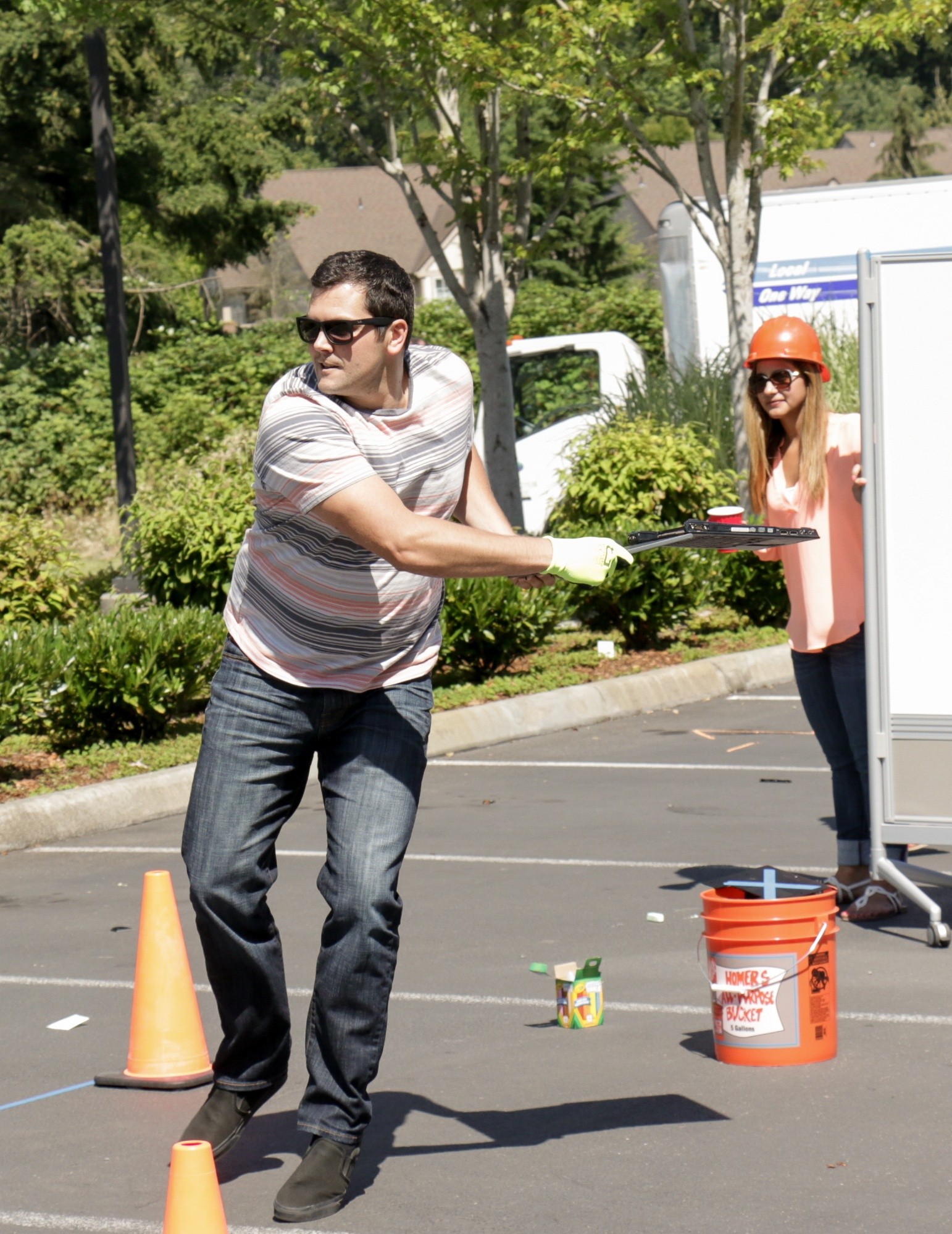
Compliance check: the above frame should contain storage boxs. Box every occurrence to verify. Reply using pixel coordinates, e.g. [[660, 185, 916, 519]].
[[552, 957, 605, 1030]]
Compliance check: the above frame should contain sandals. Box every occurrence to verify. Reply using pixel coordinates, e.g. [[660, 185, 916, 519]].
[[822, 874, 873, 904], [841, 886, 910, 921]]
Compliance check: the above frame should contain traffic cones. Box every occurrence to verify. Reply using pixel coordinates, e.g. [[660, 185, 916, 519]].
[[161, 1139, 231, 1234], [93, 867, 215, 1089]]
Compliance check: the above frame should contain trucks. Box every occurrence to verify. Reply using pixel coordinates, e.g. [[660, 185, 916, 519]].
[[659, 172, 949, 469]]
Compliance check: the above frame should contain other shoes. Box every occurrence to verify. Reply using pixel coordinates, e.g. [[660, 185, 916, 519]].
[[178, 1074, 288, 1162], [273, 1136, 361, 1223]]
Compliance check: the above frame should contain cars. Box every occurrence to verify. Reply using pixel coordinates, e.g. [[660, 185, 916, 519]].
[[466, 330, 650, 539]]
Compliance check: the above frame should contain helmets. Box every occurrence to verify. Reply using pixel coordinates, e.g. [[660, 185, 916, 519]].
[[743, 315, 831, 383]]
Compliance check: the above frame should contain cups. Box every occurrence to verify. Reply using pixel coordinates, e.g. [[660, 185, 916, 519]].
[[707, 506, 745, 554]]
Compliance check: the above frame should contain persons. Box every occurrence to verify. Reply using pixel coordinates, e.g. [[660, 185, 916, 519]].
[[741, 315, 909, 924], [179, 248, 633, 1223]]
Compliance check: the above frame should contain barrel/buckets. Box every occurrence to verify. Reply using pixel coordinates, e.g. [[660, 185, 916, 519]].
[[697, 886, 841, 1067]]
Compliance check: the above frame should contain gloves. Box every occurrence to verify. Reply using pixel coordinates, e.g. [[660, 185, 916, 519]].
[[539, 535, 634, 586]]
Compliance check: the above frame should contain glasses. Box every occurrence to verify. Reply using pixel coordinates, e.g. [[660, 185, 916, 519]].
[[748, 368, 803, 395], [295, 315, 391, 346]]
